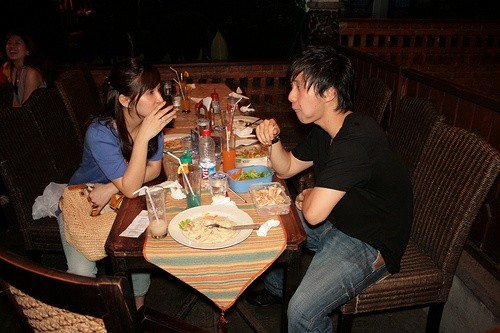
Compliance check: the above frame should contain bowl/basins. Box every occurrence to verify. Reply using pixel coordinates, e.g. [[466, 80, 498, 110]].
[[249, 181, 291, 216], [227, 165, 275, 193]]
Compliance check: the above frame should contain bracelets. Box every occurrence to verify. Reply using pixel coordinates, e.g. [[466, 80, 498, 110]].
[[272, 135, 280, 144]]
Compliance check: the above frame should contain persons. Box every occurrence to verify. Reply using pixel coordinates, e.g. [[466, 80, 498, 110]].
[[256, 43, 414, 333], [0, 32, 47, 106], [56, 58, 177, 310]]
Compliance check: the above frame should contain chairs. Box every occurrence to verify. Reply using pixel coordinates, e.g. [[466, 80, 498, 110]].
[[0, 68, 208, 333], [280, 78, 500, 333]]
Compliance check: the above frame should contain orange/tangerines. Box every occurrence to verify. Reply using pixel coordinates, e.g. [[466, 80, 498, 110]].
[[177, 163, 189, 174]]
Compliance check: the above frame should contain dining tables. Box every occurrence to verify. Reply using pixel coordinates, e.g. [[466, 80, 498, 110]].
[[104, 82, 307, 333]]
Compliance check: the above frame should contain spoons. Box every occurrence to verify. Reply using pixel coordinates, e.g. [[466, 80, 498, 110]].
[[206, 223, 260, 230]]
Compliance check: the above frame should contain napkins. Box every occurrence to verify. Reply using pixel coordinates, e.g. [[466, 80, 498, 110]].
[[234, 127, 256, 137], [234, 139, 258, 148], [228, 92, 250, 100], [256, 220, 280, 237], [138, 180, 187, 200], [240, 103, 255, 112]]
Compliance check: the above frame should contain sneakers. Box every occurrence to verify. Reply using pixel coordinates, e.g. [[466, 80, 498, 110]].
[[246, 286, 284, 308]]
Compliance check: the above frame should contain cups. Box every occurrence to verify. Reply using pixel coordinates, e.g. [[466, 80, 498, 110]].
[[198, 118, 209, 135], [175, 72, 186, 94], [145, 186, 168, 239], [208, 171, 228, 197], [220, 109, 235, 130], [179, 86, 191, 114], [181, 165, 203, 208], [219, 127, 235, 172]]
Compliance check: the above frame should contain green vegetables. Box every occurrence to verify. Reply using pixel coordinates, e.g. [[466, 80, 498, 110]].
[[179, 218, 190, 228], [235, 169, 271, 181]]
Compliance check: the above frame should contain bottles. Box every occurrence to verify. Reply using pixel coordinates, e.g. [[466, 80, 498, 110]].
[[163, 82, 172, 96], [211, 89, 219, 104], [198, 129, 217, 181], [173, 96, 182, 114], [178, 148, 195, 188]]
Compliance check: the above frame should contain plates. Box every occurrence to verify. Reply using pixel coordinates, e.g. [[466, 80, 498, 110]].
[[232, 116, 263, 137], [163, 133, 191, 153], [229, 138, 269, 159], [167, 204, 255, 248]]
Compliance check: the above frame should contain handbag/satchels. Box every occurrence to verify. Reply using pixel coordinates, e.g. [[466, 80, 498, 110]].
[[59, 183, 124, 261]]
